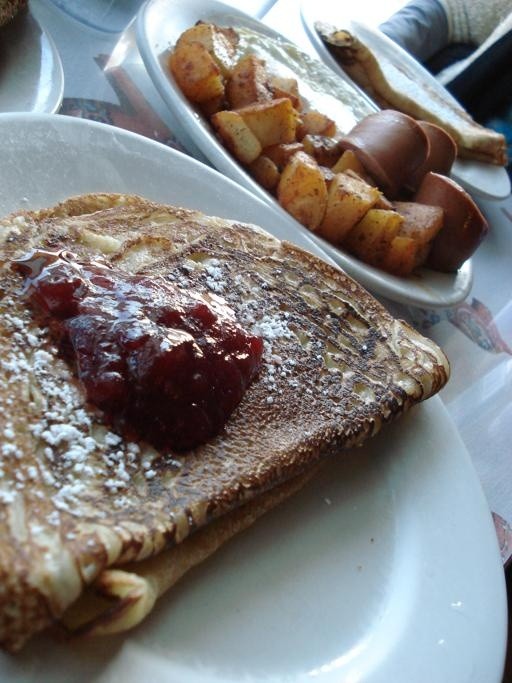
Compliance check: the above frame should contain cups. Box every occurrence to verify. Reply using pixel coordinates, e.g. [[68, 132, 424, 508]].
[[52, 0, 148, 41]]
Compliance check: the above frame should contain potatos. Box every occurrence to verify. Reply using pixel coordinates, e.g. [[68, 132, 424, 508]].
[[170, 20, 444, 274]]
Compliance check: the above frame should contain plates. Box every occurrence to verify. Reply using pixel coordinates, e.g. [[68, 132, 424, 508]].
[[2, 0, 67, 113], [134, 0, 474, 311], [2, 112, 511, 681], [294, 2, 511, 202]]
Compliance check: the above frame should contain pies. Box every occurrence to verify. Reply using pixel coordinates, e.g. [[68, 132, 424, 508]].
[[0, 190, 454, 657], [314, 16, 507, 164]]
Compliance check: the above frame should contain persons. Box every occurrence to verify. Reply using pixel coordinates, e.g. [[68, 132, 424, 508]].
[[375, 1, 510, 166]]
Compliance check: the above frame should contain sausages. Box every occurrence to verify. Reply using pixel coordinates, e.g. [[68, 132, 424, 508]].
[[336, 107, 490, 274]]
